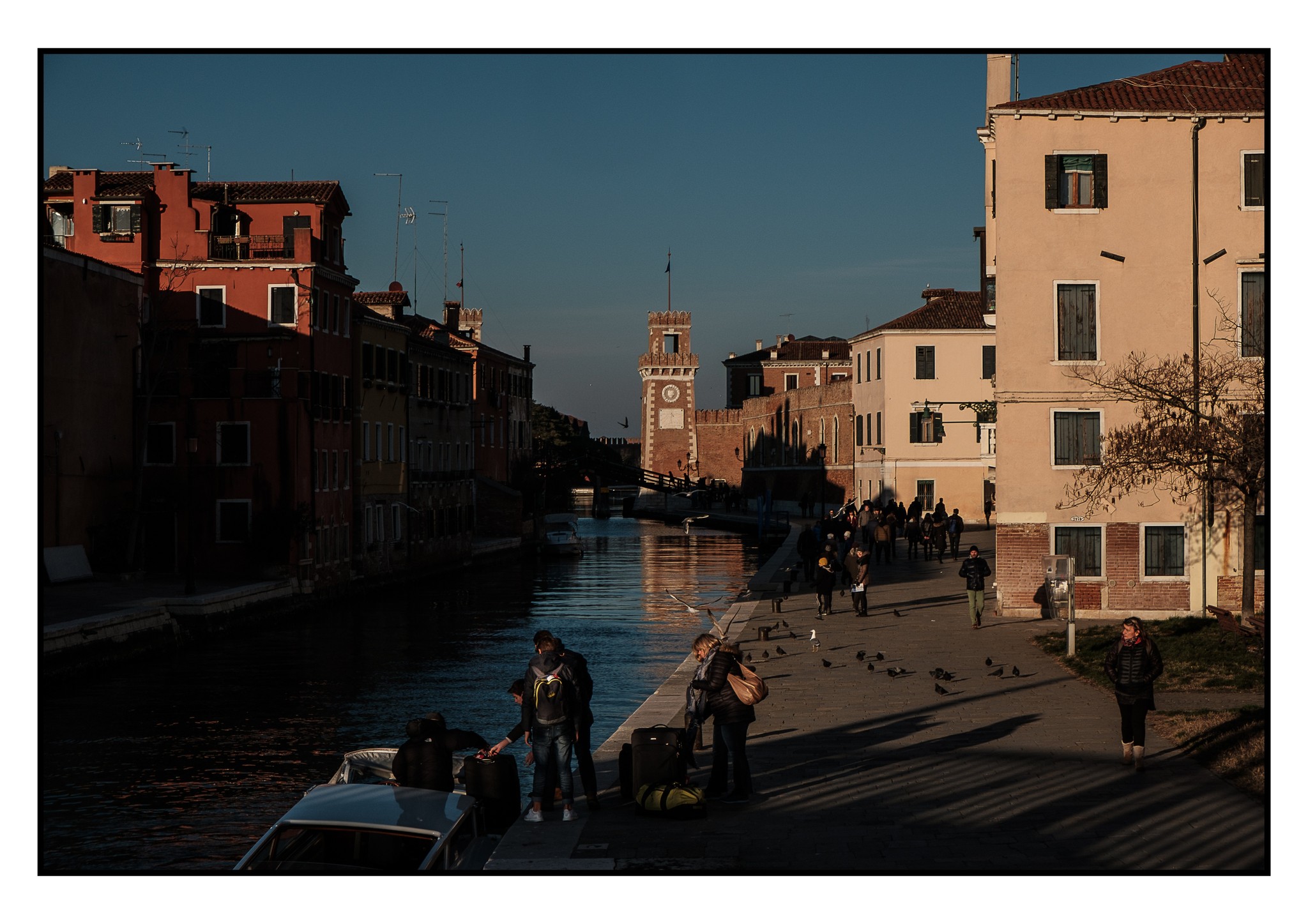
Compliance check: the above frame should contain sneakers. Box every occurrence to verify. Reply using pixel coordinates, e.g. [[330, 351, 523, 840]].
[[524, 808, 543, 822], [563, 809, 579, 821]]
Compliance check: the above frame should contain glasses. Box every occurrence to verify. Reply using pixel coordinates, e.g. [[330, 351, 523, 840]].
[[1125, 618, 1138, 630]]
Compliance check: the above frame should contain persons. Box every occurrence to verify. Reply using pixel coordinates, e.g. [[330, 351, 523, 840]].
[[488, 630, 601, 823], [683, 473, 691, 492], [958, 546, 992, 629], [684, 633, 756, 804], [1104, 617, 1163, 773], [668, 471, 677, 489], [796, 492, 964, 620], [984, 496, 992, 531], [391, 712, 492, 794], [698, 477, 749, 516], [765, 495, 774, 516]]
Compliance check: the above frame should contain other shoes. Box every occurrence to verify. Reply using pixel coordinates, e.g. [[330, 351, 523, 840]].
[[856, 611, 867, 617], [701, 788, 721, 799], [587, 795, 599, 807], [953, 557, 958, 561], [822, 610, 828, 616], [827, 610, 831, 614], [975, 616, 982, 626], [720, 793, 750, 803], [972, 625, 977, 629], [853, 608, 861, 613]]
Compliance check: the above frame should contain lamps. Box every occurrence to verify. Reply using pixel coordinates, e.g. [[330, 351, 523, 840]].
[[938, 421, 994, 437], [861, 447, 885, 456], [921, 400, 997, 424]]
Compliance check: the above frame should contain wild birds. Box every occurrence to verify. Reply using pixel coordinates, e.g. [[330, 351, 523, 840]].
[[810, 629, 821, 652], [707, 605, 741, 642], [617, 416, 629, 429], [665, 588, 723, 614], [672, 489, 706, 496], [682, 515, 709, 534]]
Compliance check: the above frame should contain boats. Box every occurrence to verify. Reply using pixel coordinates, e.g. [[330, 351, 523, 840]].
[[544, 521, 579, 555], [233, 748, 487, 876]]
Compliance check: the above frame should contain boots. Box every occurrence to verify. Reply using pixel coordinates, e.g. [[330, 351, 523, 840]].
[[1132, 744, 1146, 775], [1118, 740, 1134, 769]]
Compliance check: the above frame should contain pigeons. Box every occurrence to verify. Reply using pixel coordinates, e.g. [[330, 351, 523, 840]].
[[783, 594, 788, 600], [744, 644, 787, 663], [770, 621, 779, 632], [821, 658, 831, 668], [789, 631, 797, 641], [928, 668, 953, 683], [894, 609, 901, 617], [987, 666, 1005, 679], [934, 683, 949, 697], [985, 656, 991, 668], [839, 589, 844, 597], [1012, 665, 1020, 679], [856, 651, 905, 679], [782, 620, 789, 629]]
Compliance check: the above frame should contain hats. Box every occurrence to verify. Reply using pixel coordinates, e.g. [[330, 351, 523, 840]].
[[426, 712, 445, 723], [819, 557, 828, 566], [857, 547, 865, 553], [844, 531, 851, 538], [970, 545, 979, 552]]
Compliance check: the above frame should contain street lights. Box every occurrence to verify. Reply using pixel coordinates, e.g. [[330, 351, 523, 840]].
[[677, 452, 700, 478], [818, 443, 827, 521]]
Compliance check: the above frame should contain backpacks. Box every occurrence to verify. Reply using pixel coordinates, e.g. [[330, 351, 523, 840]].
[[531, 665, 567, 725]]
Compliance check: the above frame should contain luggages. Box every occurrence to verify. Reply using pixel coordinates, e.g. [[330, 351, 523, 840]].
[[631, 723, 680, 798], [463, 749, 521, 836]]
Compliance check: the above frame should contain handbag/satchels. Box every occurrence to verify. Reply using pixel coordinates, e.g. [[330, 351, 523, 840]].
[[726, 656, 770, 705], [635, 782, 705, 811]]
